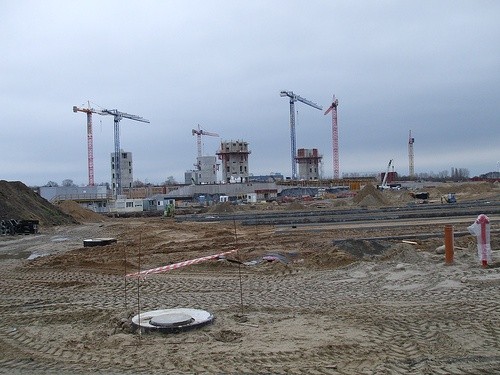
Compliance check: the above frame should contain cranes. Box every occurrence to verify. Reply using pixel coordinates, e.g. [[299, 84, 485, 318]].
[[100, 107, 150, 201], [280, 88, 323, 182], [191, 123, 220, 159], [407, 129, 415, 179], [322, 94, 340, 180], [72, 100, 108, 186]]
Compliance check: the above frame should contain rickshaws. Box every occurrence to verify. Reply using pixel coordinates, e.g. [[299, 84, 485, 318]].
[[448, 195, 456, 203]]
